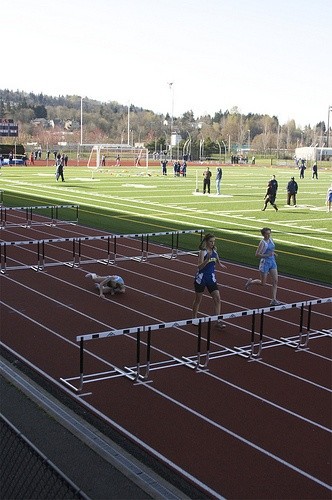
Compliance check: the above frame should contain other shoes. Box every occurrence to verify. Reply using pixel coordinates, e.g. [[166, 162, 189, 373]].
[[85, 272, 96, 278], [95, 283, 100, 288]]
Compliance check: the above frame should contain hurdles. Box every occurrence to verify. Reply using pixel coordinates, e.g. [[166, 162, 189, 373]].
[[1, 204, 80, 230], [59, 297, 332, 392], [0, 228, 205, 274]]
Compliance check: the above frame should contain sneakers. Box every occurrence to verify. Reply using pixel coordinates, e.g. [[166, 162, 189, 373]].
[[270, 300, 279, 305], [214, 322, 226, 328], [246, 278, 252, 289], [192, 322, 199, 326]]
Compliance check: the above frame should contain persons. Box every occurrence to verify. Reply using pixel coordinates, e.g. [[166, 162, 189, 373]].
[[286, 177, 298, 207], [174, 161, 187, 178], [0, 149, 69, 182], [230, 154, 255, 164], [214, 167, 222, 195], [261, 175, 278, 212], [161, 159, 168, 176], [202, 167, 211, 194], [101, 153, 121, 166], [85, 272, 126, 300], [327, 184, 332, 214], [188, 232, 228, 329], [243, 226, 285, 305], [296, 158, 318, 180]]
